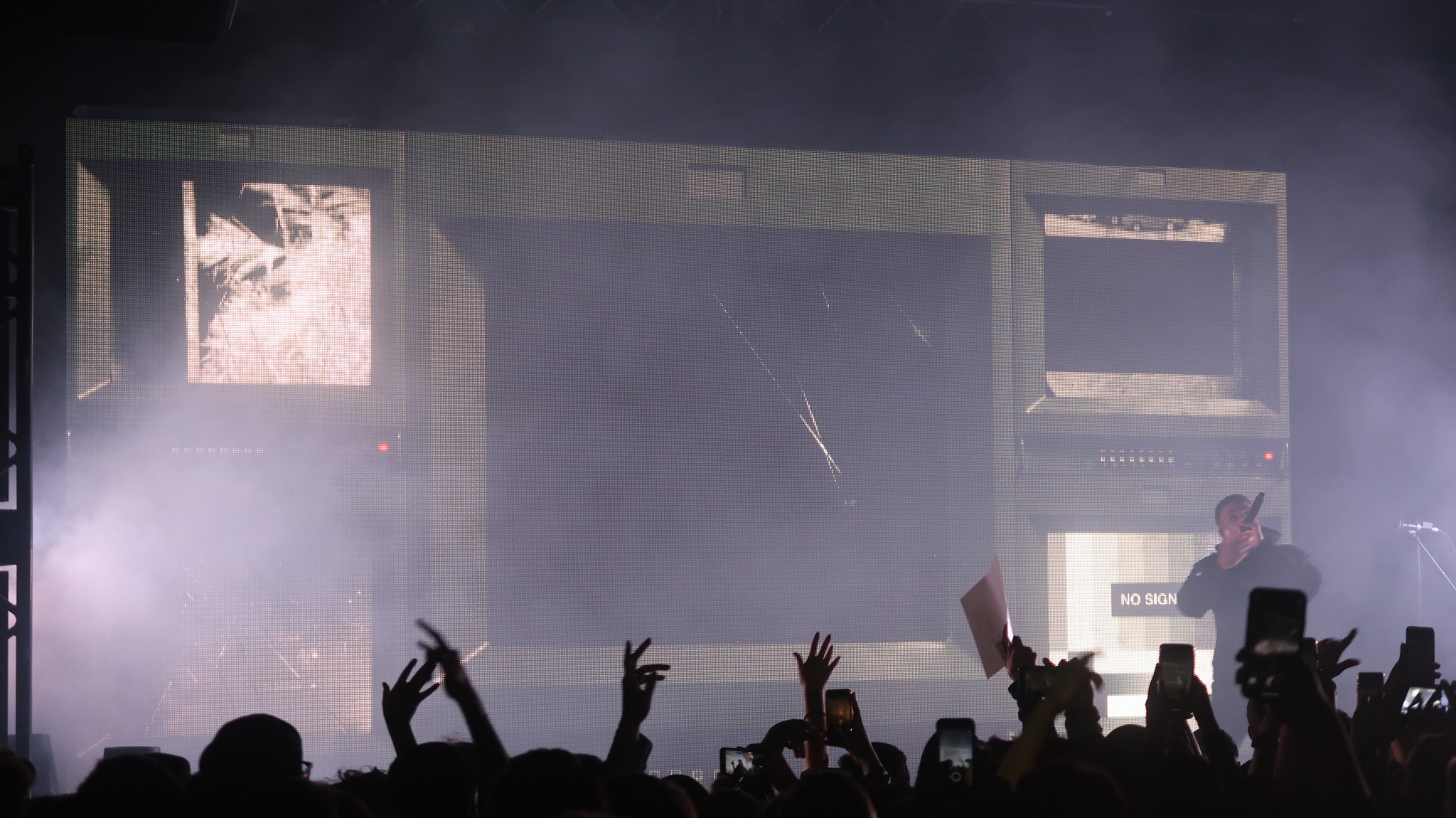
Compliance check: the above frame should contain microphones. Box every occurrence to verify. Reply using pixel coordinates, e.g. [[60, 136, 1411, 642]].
[[1240, 492, 1265, 532]]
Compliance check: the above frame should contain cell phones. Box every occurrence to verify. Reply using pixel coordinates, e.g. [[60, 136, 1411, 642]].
[[1405, 625, 1435, 686], [1017, 665, 1060, 696], [935, 716, 975, 771], [1244, 587, 1306, 681], [825, 688, 853, 745], [1159, 643, 1195, 702], [1356, 671, 1385, 703], [719, 747, 756, 778]]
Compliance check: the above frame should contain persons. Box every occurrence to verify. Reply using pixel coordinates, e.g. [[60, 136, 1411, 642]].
[[198, 620, 1456, 818], [1177, 494, 1323, 746]]
